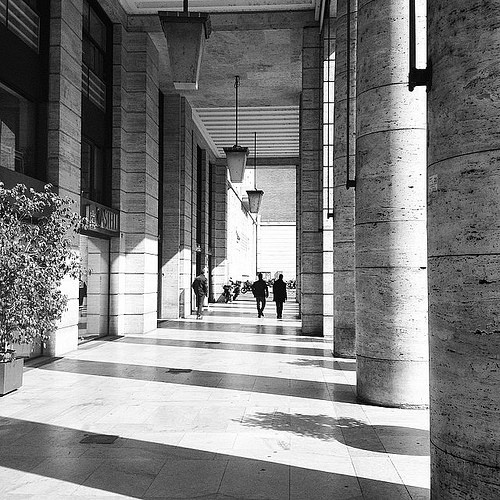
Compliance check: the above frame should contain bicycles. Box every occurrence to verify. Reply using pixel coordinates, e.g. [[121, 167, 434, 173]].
[[222, 279, 241, 302], [233, 278, 298, 297]]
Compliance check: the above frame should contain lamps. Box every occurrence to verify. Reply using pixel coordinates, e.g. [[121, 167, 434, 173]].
[[223, 75, 250, 184], [246, 132, 265, 213], [158, 0, 213, 91]]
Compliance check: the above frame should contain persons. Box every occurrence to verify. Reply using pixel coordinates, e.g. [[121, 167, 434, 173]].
[[251, 273, 269, 318], [273, 273, 287, 319], [192, 271, 208, 319]]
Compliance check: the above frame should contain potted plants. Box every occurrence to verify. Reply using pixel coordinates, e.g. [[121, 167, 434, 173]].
[[1, 181, 94, 397]]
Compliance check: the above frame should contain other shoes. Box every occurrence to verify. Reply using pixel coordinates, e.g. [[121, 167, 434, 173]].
[[258, 316, 261, 318], [259, 309, 264, 316], [197, 315, 203, 320]]
